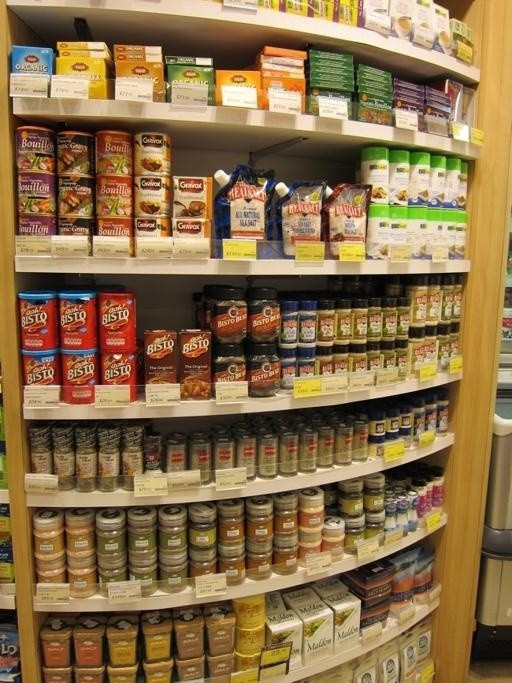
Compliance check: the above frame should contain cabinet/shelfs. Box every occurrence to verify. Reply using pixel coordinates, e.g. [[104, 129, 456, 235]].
[[0, 0, 512, 683]]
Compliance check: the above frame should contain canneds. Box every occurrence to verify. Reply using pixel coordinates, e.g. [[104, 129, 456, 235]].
[[34, 474, 387, 598]]
